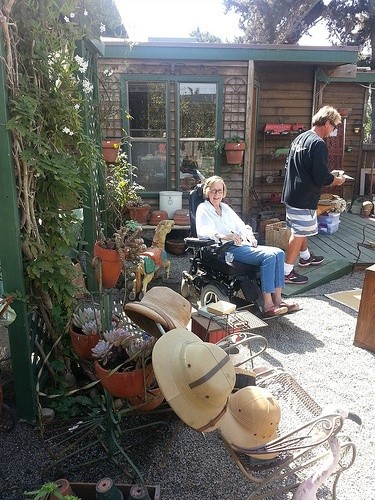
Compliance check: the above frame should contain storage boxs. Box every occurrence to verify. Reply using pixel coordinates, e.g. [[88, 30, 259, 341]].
[[40, 482, 160, 500], [316, 213, 342, 234], [191, 313, 242, 344]]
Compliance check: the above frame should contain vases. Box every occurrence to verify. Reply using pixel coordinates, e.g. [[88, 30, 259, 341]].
[[151, 210, 191, 254], [102, 140, 123, 164]]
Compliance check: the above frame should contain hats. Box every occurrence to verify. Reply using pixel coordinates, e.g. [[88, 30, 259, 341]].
[[124, 284, 192, 336], [217, 385, 281, 448], [152, 328, 236, 432]]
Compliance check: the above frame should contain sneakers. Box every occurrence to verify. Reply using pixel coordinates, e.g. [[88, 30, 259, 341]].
[[299, 252, 325, 266], [284, 269, 308, 283]]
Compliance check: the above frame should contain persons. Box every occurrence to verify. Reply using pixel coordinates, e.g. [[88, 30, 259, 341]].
[[195, 175, 300, 317], [282, 106, 346, 284]]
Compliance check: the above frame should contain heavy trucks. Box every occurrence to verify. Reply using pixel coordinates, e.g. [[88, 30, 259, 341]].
[[264, 122, 306, 134]]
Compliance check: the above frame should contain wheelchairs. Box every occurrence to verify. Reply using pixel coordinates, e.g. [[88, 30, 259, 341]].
[[182, 183, 262, 310]]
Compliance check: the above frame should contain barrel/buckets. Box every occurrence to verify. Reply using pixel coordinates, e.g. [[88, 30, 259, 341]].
[[159, 190, 183, 219]]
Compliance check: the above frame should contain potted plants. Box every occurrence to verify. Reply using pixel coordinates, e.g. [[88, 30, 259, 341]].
[[70, 308, 164, 411], [126, 183, 153, 225], [216, 135, 248, 166], [93, 219, 147, 290]]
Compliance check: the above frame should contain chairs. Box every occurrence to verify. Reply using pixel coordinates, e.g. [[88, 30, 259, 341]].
[[155, 321, 356, 500]]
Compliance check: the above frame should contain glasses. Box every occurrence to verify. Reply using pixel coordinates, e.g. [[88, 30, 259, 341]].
[[211, 189, 224, 194], [331, 121, 337, 130]]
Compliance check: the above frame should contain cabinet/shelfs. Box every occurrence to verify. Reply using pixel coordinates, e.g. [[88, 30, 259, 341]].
[[262, 132, 299, 185]]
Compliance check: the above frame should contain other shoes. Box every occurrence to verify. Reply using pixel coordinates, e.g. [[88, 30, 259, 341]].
[[280, 302, 299, 311], [262, 306, 288, 318]]
